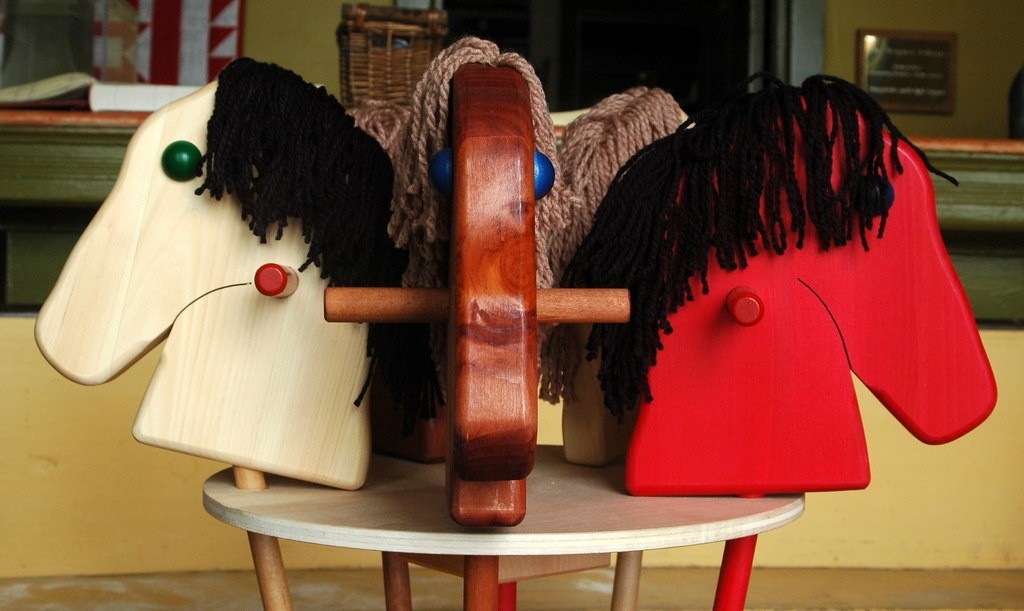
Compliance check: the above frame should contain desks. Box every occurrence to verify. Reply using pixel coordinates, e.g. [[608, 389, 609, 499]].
[[199, 443, 802, 611]]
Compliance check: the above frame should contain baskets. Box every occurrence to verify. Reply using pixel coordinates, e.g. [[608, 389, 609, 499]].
[[335, 11, 442, 108]]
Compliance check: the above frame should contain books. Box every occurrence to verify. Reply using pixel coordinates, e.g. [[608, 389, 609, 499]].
[[0, 69, 204, 112]]
